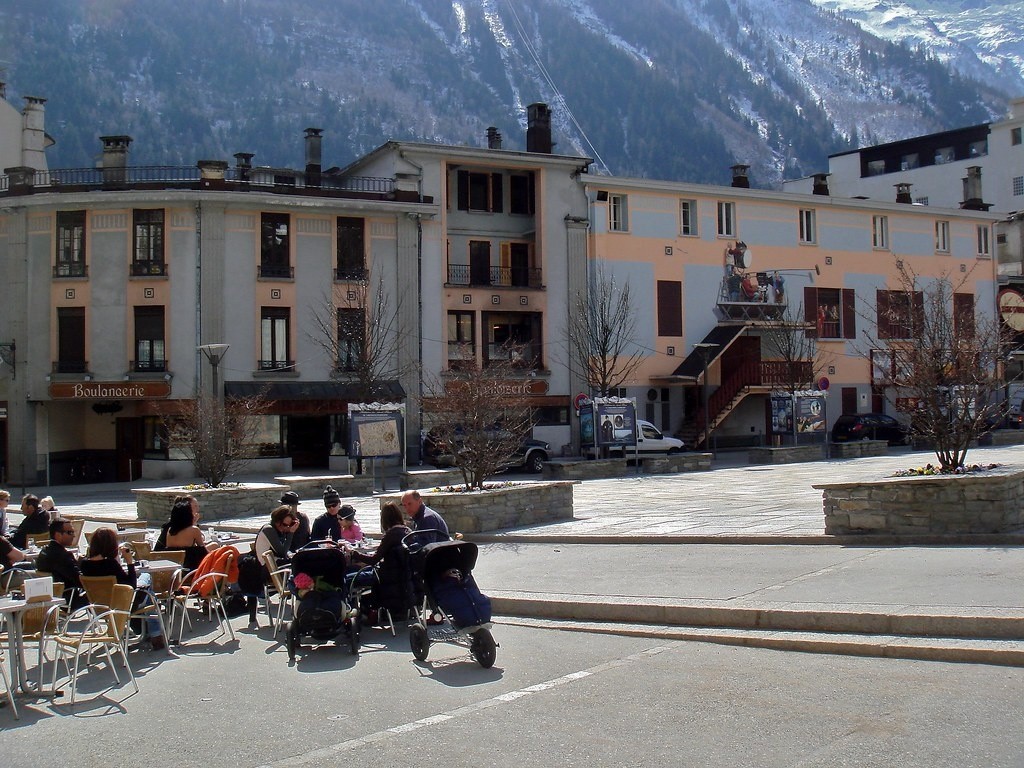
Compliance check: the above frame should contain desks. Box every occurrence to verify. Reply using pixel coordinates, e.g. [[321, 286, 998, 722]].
[[22, 546, 81, 559], [0, 592, 64, 706], [121, 558, 183, 650], [145, 532, 256, 606]]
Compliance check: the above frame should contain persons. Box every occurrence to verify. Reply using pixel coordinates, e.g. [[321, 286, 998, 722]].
[[0, 490, 449, 649], [726, 241, 785, 303], [819, 304, 841, 337]]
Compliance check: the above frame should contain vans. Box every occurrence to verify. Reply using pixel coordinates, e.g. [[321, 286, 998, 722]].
[[585, 419, 686, 460]]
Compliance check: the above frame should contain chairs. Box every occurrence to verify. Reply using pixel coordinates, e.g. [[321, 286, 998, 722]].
[[0, 514, 422, 720]]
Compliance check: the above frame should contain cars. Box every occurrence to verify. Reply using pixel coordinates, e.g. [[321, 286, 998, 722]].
[[830, 413, 913, 446], [984, 411, 1023, 428]]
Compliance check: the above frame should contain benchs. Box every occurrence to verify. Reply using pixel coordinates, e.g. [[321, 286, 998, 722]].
[[543, 458, 627, 481], [830, 439, 889, 460], [910, 434, 977, 452], [274, 476, 375, 499], [397, 468, 474, 491], [644, 452, 713, 473], [979, 429, 1024, 445]]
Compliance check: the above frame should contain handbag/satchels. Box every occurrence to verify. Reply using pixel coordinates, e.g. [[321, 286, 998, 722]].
[[219, 593, 249, 618]]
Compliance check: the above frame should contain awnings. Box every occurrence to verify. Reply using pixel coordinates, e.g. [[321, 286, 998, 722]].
[[224, 381, 407, 414]]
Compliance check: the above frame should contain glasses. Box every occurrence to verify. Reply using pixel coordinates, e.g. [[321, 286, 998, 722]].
[[326, 502, 338, 508], [337, 513, 353, 521], [62, 531, 75, 535], [282, 523, 292, 527]]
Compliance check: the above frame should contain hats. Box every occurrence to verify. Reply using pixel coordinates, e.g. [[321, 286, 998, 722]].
[[323, 485, 339, 502], [278, 492, 302, 506], [337, 505, 354, 521]]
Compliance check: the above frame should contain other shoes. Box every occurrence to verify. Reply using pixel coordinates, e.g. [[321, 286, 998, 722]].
[[203, 603, 215, 614], [426, 612, 444, 626], [128, 636, 149, 651], [152, 635, 179, 650]]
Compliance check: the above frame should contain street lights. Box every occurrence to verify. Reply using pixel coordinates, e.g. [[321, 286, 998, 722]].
[[692, 343, 720, 451], [194, 343, 231, 453]]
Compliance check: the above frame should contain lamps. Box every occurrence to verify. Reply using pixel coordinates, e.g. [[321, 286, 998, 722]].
[[164, 373, 174, 380], [84, 375, 93, 381], [124, 372, 130, 380], [45, 375, 51, 382]]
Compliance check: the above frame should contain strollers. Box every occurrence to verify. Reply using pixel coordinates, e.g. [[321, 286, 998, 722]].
[[285, 541, 361, 663], [400, 528, 496, 669]]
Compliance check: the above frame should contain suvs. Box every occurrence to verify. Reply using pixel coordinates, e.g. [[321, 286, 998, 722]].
[[422, 422, 552, 473]]
